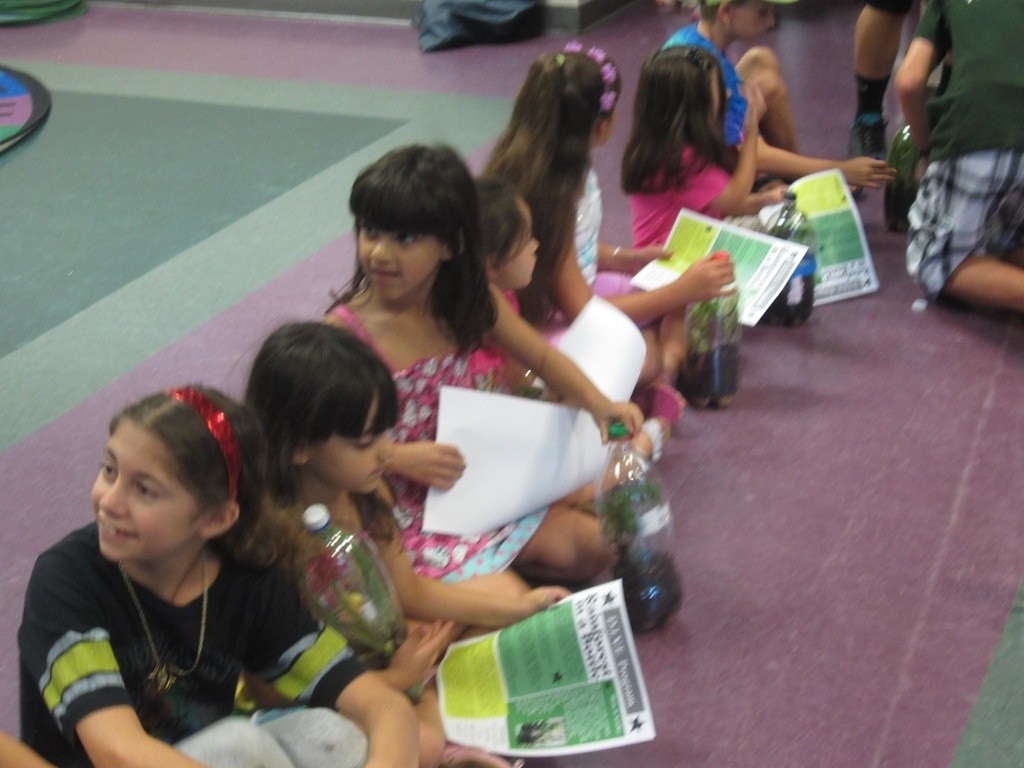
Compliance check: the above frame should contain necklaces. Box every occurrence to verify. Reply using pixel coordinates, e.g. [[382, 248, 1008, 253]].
[[118, 545, 207, 692]]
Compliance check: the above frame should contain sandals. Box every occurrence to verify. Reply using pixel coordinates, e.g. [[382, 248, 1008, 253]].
[[638, 414, 667, 469]]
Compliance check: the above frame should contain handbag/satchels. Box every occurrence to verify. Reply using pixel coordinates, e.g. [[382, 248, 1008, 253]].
[[414, 0, 546, 52]]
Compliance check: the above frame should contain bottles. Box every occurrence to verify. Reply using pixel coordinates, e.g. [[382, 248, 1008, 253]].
[[762, 192, 816, 329], [293, 504, 425, 705], [884, 124, 921, 233], [594, 421, 686, 633], [684, 251, 744, 407]]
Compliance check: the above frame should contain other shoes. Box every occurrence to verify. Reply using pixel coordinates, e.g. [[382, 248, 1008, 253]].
[[638, 384, 684, 423]]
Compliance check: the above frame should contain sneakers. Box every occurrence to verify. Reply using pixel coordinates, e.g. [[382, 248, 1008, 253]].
[[852, 110, 889, 163]]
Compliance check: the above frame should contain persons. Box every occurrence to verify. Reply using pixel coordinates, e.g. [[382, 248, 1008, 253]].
[[845, 0, 952, 158], [17, 385, 420, 768], [245, 321, 572, 768], [894, 0, 1024, 309], [619, 45, 791, 249], [323, 143, 643, 598], [481, 40, 738, 421], [474, 174, 671, 465], [670, 0, 898, 197]]
